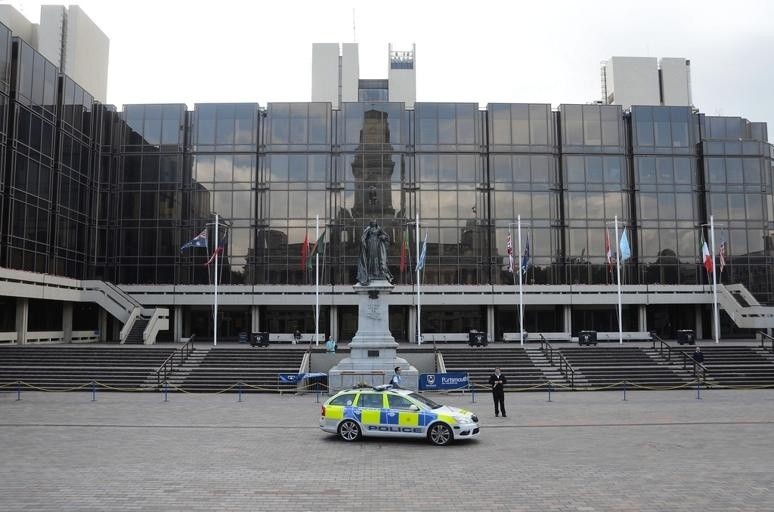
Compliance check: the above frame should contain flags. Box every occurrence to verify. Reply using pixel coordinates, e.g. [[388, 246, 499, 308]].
[[299, 234, 308, 272], [522, 232, 529, 275], [307, 230, 325, 269], [604, 227, 613, 273], [507, 232, 515, 273], [414, 230, 428, 272], [180, 228, 207, 254], [699, 228, 713, 273], [399, 227, 411, 272], [619, 227, 631, 261], [203, 235, 225, 265], [718, 240, 726, 273]]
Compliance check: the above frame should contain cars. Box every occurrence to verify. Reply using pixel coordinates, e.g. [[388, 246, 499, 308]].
[[318, 383, 481, 446]]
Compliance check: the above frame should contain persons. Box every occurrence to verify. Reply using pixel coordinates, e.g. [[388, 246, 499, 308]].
[[293, 329, 301, 343], [523, 329, 528, 343], [392, 367, 406, 389], [693, 347, 705, 372], [356, 217, 395, 286], [326, 335, 336, 355], [489, 367, 507, 417]]
